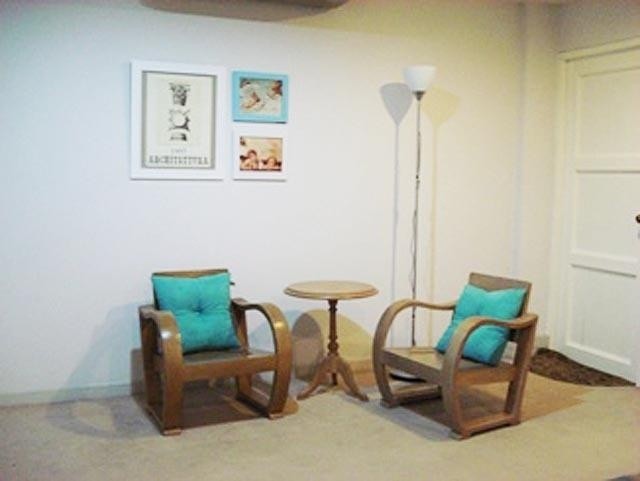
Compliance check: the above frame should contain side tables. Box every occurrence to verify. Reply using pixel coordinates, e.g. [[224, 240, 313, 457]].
[[283, 281, 379, 402]]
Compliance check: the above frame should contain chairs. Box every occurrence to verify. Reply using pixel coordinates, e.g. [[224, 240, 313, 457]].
[[137, 269, 294, 436], [371, 272, 539, 438]]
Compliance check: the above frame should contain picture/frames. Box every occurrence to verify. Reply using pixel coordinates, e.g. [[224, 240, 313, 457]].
[[231, 70, 289, 124], [234, 131, 288, 182], [131, 60, 226, 180]]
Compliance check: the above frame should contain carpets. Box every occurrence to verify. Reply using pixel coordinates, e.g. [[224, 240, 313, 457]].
[[0, 363, 640, 481]]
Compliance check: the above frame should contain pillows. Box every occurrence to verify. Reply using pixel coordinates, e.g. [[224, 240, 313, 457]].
[[434, 283, 528, 367], [152, 272, 243, 356]]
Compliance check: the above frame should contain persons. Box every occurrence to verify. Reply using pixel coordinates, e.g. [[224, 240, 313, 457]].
[[262, 155, 280, 170], [240, 80, 260, 108], [271, 80, 282, 97], [241, 150, 260, 170]]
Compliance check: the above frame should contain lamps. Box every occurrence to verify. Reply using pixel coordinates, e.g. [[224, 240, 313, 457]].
[[388, 63, 432, 383]]
